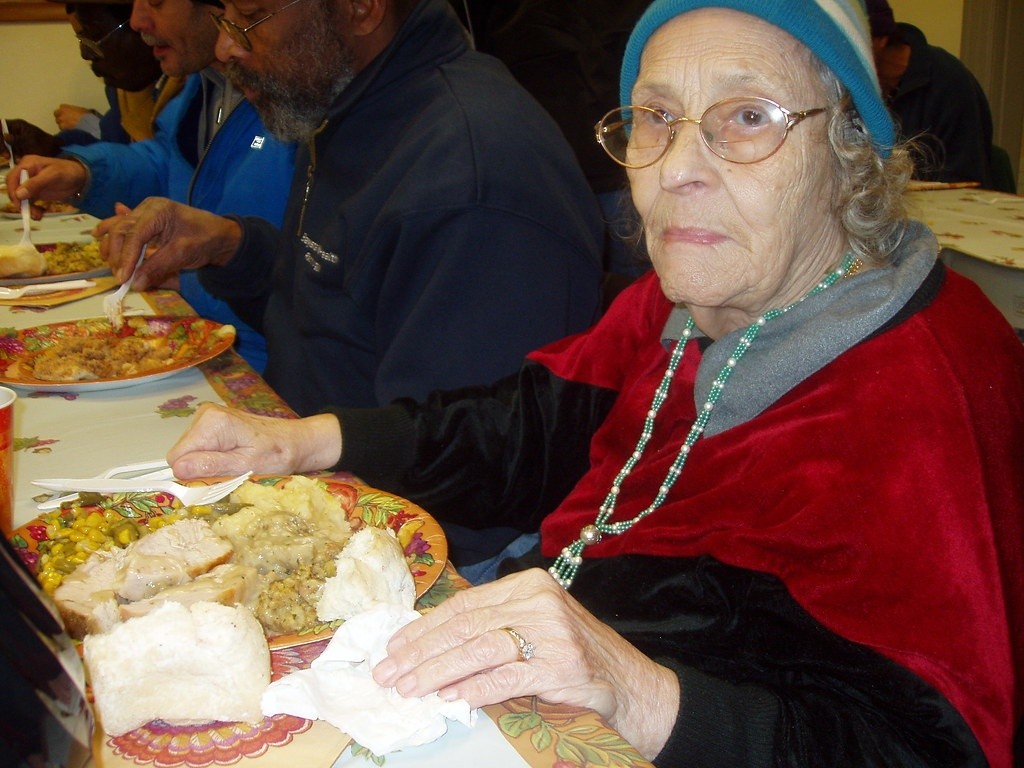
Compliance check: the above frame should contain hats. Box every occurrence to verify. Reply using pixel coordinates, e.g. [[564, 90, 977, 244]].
[[618, 0, 899, 161]]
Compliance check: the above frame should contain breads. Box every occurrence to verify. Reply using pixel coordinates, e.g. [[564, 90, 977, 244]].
[[314, 526, 418, 621], [83, 601, 273, 737], [0, 244, 47, 276]]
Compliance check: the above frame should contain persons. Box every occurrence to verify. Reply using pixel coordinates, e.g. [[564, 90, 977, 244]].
[[867, 0, 994, 190], [167, 0, 1024, 768], [0, 0, 606, 591]]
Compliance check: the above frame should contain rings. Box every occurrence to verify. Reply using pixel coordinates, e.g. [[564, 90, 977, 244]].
[[501, 627, 536, 662]]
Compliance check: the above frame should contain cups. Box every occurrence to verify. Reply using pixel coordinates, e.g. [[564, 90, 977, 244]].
[[0, 385, 18, 537]]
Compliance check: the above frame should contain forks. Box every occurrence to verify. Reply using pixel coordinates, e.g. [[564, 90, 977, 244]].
[[103, 242, 147, 327], [0, 279, 95, 300], [29, 468, 253, 508]]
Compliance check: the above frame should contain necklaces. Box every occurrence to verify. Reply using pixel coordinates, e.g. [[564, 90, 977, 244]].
[[548, 250, 857, 586], [842, 258, 863, 280]]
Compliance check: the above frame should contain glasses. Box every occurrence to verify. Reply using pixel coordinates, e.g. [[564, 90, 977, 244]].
[[208, 0, 301, 52], [65, 17, 131, 60], [593, 95, 850, 170]]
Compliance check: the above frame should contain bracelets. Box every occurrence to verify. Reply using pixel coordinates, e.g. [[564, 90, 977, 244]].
[[69, 157, 88, 199]]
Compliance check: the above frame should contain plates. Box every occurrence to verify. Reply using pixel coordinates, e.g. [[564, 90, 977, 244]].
[[0, 241, 111, 282], [0, 208, 82, 221], [0, 544, 96, 749], [0, 315, 236, 393], [6, 474, 446, 655]]
